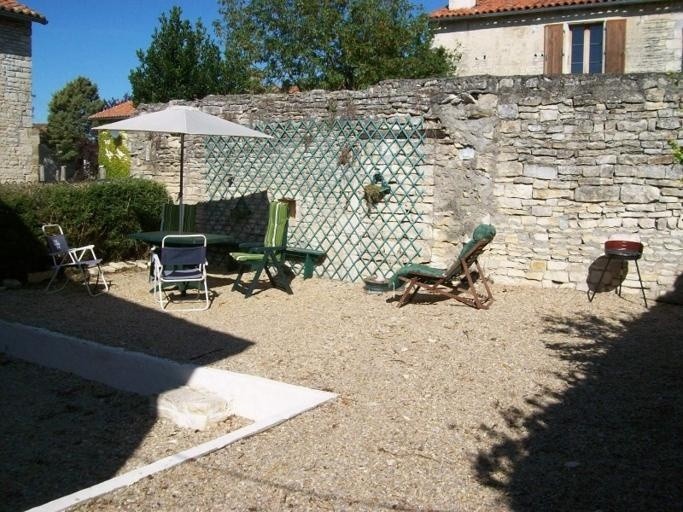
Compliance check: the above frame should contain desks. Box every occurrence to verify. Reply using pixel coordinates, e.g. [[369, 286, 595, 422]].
[[127, 230, 236, 290]]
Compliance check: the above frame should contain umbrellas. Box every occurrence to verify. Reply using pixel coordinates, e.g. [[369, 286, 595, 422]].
[[90, 104, 276, 231]]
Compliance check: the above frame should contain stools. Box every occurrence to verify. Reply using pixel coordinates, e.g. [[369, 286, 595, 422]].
[[590, 239, 648, 309]]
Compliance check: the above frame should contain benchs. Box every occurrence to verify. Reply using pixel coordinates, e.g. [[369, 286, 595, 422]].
[[238, 242, 327, 280]]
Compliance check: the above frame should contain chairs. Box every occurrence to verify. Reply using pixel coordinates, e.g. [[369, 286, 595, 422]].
[[41, 223, 108, 296], [387, 224, 496, 310], [148, 203, 211, 314], [228, 201, 294, 298]]
